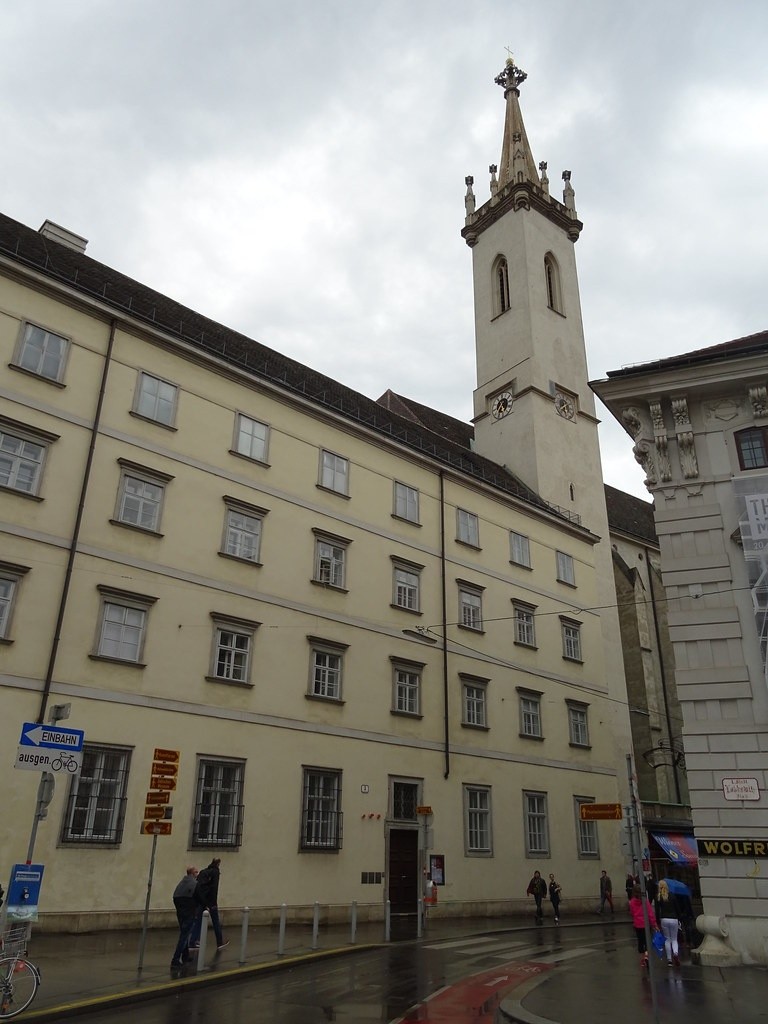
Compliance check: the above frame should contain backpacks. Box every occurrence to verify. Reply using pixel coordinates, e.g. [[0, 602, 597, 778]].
[[197, 866, 214, 895]]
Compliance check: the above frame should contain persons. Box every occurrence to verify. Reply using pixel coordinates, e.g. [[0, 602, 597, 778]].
[[189, 857, 230, 950], [654, 880, 684, 967], [548, 873, 563, 922], [625, 874, 634, 900], [527, 870, 547, 923], [628, 884, 660, 967], [170, 864, 198, 969], [597, 870, 614, 914]]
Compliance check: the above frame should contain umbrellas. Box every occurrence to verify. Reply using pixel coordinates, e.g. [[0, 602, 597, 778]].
[[651, 929, 668, 961], [656, 878, 691, 896]]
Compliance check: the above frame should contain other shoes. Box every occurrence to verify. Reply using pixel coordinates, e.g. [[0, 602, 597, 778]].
[[554, 917, 558, 922], [640, 959, 645, 966], [183, 958, 193, 962], [645, 956, 649, 965], [673, 954, 681, 966], [188, 945, 199, 950], [667, 960, 673, 967], [170, 962, 182, 969], [217, 940, 230, 948]]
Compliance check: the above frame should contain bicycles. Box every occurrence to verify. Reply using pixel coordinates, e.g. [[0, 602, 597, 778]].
[[0, 923, 43, 1019]]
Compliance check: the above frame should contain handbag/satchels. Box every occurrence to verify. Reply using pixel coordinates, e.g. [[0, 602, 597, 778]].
[[534, 882, 542, 895]]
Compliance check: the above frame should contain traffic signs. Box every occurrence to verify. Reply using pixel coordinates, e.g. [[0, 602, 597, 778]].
[[18, 723, 85, 753]]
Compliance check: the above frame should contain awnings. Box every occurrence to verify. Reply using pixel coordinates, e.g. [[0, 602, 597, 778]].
[[651, 832, 698, 867]]
